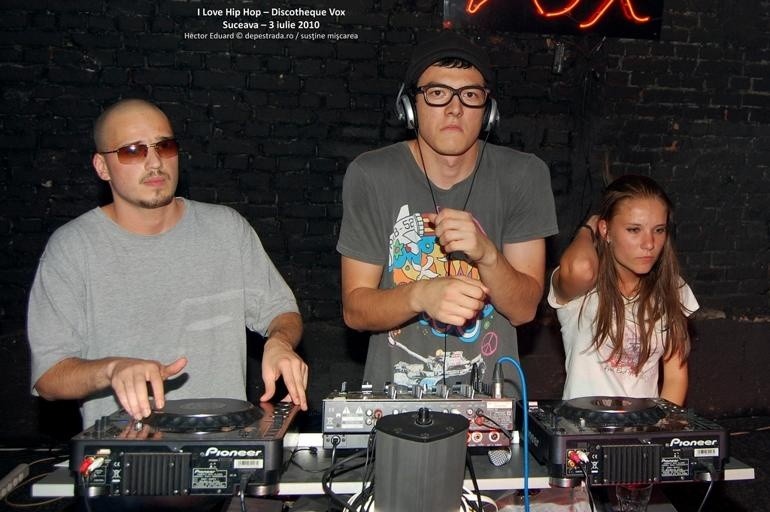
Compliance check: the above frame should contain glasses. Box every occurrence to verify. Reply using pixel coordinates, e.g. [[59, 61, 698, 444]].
[[411, 84, 490, 110], [101, 136, 181, 166]]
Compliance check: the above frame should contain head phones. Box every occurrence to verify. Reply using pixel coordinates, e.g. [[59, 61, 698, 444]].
[[393, 50, 500, 133]]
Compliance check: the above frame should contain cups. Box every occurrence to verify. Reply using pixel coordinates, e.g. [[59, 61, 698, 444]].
[[615, 484, 653, 512]]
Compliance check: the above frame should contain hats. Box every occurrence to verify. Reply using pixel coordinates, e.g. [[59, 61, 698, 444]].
[[403, 31, 493, 90]]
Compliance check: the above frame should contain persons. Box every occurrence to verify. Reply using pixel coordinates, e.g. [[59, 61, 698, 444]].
[[25, 98, 309, 434], [546, 173, 700, 408], [335, 27, 560, 406]]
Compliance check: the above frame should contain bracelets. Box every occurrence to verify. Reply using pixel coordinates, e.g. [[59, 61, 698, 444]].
[[581, 224, 594, 244]]
